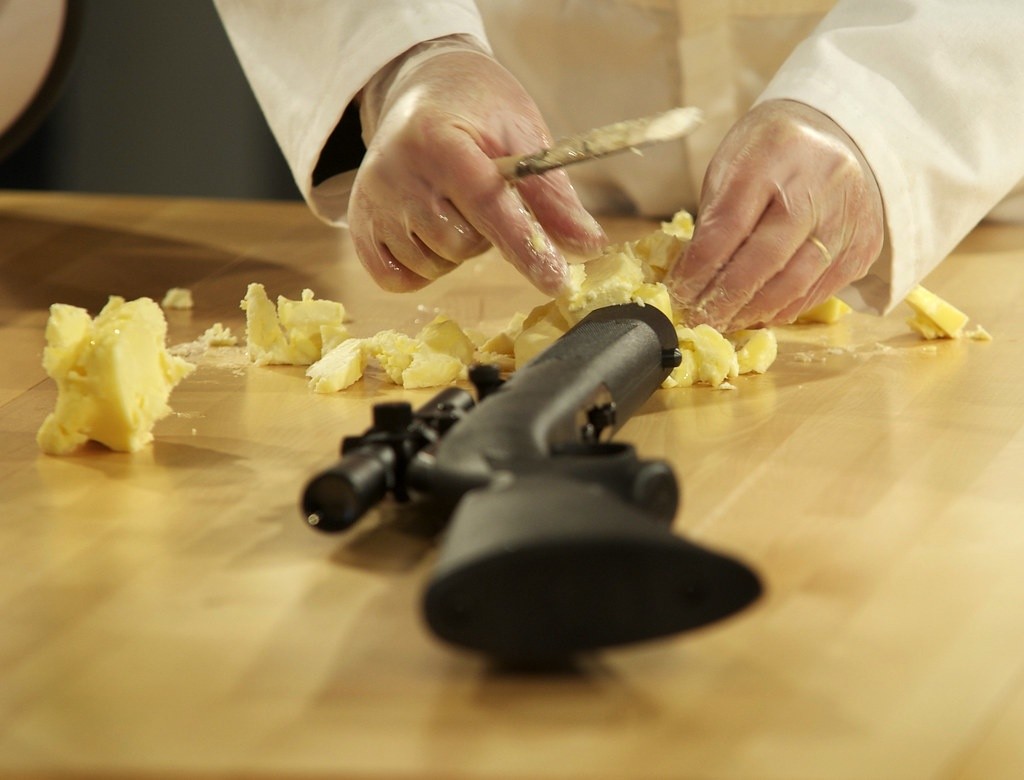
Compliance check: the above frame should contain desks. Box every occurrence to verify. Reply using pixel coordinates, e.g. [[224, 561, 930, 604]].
[[0, 189, 1024, 780]]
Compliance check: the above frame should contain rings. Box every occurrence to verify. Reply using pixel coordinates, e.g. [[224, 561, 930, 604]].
[[808, 235, 833, 264]]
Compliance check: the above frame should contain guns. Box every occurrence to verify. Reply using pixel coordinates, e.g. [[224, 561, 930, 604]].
[[298, 299, 770, 664]]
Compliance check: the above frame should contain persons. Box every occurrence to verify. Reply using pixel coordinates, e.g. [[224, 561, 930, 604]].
[[213, 0, 1024, 338]]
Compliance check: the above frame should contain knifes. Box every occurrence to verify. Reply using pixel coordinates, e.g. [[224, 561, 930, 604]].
[[490, 107, 700, 181]]
